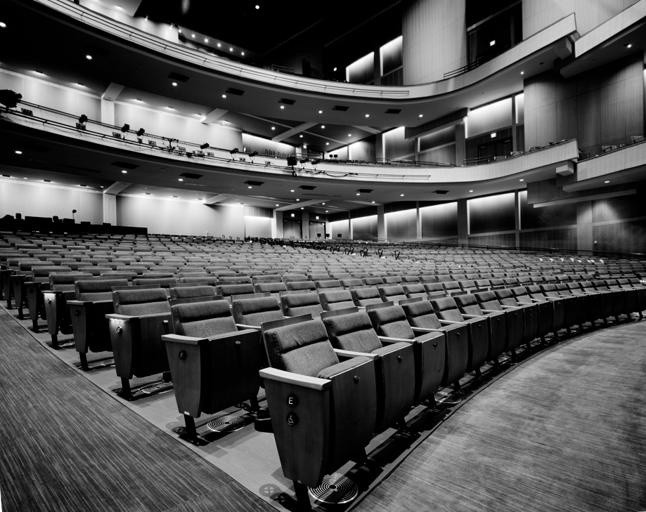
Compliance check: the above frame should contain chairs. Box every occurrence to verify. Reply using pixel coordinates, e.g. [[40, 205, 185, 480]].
[[494, 133, 644, 161]]
